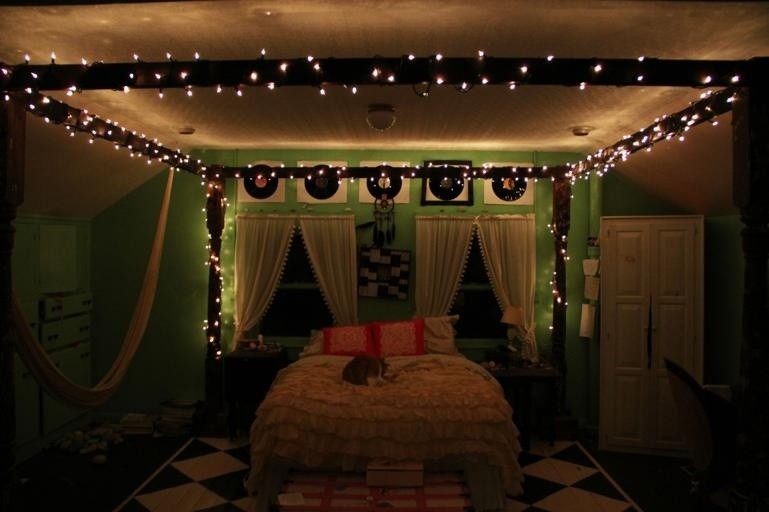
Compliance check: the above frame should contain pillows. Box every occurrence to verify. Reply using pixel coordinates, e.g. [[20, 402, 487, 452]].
[[322, 324, 373, 357], [423, 315, 460, 355], [297, 330, 324, 356], [372, 318, 427, 357]]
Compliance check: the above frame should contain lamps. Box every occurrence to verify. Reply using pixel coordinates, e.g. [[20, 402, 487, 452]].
[[366, 104, 397, 132], [500, 305, 525, 365]]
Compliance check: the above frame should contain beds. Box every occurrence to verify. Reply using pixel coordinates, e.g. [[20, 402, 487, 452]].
[[243, 314, 526, 510]]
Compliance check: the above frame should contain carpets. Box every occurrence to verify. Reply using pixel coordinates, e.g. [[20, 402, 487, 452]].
[[110, 435, 645, 512]]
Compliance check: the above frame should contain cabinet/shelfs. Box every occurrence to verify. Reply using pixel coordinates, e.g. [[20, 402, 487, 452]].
[[11, 214, 95, 467], [598, 215, 705, 458]]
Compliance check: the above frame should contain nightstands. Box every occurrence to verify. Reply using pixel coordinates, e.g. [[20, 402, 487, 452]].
[[223, 347, 288, 432], [488, 357, 565, 455]]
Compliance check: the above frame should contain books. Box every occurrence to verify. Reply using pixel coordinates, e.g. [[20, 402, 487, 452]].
[[116, 407, 201, 435]]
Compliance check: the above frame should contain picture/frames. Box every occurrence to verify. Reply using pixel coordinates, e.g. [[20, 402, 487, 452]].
[[421, 159, 474, 206]]
